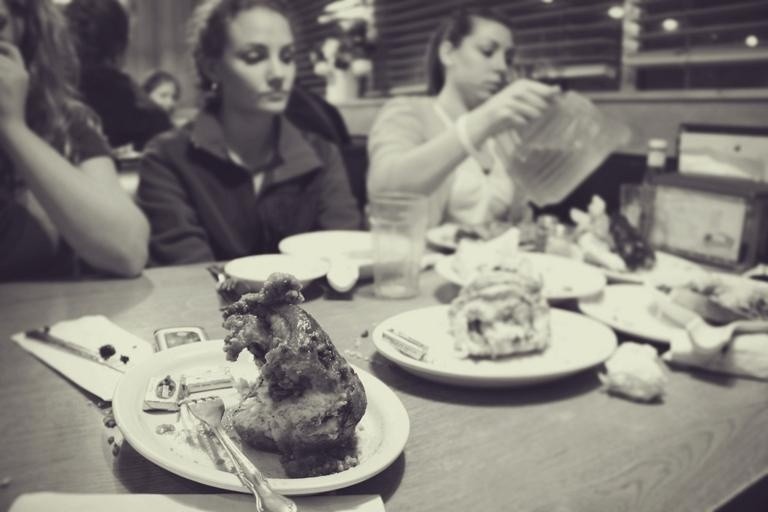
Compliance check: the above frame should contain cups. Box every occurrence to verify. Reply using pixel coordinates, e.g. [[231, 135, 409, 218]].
[[368, 189, 426, 298], [503, 91, 631, 208]]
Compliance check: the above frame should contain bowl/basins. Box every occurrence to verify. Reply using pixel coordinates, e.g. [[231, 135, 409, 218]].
[[224, 253, 330, 289]]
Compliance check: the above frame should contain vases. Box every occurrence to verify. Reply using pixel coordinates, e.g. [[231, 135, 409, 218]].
[[323, 67, 360, 106]]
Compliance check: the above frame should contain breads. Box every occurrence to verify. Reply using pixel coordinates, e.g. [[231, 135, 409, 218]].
[[448, 260, 553, 362]]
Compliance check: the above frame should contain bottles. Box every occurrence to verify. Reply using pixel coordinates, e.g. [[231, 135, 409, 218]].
[[643, 136, 667, 171], [605, 205, 657, 273]]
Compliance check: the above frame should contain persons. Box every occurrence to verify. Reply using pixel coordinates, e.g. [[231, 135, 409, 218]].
[[366, 4, 565, 241], [60, 0, 176, 152], [137, 0, 366, 271], [142, 68, 183, 119], [0, 1, 152, 280]]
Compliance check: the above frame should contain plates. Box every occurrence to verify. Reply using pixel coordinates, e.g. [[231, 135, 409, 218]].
[[432, 248, 608, 303], [578, 240, 712, 289], [280, 228, 412, 276], [424, 219, 569, 253], [373, 298, 619, 388], [651, 269, 768, 352], [111, 339, 411, 497], [575, 282, 672, 347]]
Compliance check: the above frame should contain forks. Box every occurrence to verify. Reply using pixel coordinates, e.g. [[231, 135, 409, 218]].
[[207, 263, 240, 295], [175, 392, 299, 512]]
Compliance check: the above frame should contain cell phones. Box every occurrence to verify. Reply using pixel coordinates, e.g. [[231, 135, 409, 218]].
[[154, 324, 208, 353]]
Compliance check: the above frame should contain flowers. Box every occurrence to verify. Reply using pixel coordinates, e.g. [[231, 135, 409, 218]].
[[309, 2, 387, 74]]
